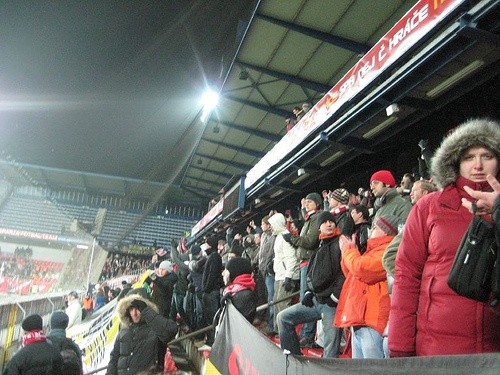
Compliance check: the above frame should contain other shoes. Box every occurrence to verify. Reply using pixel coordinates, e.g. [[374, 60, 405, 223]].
[[298, 337, 315, 348]]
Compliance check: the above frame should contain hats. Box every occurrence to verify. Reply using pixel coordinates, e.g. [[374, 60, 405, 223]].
[[51, 311, 68, 329], [207, 237, 218, 247], [160, 260, 173, 273], [332, 189, 349, 204], [225, 257, 252, 281], [192, 246, 201, 254], [317, 211, 337, 229], [369, 170, 395, 187], [376, 214, 399, 236], [22, 314, 43, 331], [306, 192, 322, 206]]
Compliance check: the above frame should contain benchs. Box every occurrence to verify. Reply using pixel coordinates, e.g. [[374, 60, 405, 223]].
[[163, 323, 214, 375], [272, 294, 352, 358]]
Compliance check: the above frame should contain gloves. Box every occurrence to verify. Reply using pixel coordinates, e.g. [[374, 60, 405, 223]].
[[327, 293, 339, 307], [283, 234, 291, 242], [301, 290, 314, 307], [131, 300, 147, 312], [205, 288, 213, 293], [282, 277, 292, 291]]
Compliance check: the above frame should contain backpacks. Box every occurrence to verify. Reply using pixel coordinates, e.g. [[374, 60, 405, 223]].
[[48, 335, 81, 375]]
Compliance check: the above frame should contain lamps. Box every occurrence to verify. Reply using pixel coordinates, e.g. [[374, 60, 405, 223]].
[[386, 103, 400, 116], [298, 168, 306, 176], [213, 124, 219, 133], [255, 198, 260, 204], [240, 66, 249, 80], [197, 156, 202, 164]]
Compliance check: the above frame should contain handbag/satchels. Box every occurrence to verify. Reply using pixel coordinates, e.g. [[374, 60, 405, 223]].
[[447, 202, 496, 304]]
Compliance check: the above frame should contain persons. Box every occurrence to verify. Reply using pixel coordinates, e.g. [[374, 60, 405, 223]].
[[0, 103, 500, 375], [387, 119, 500, 358]]
